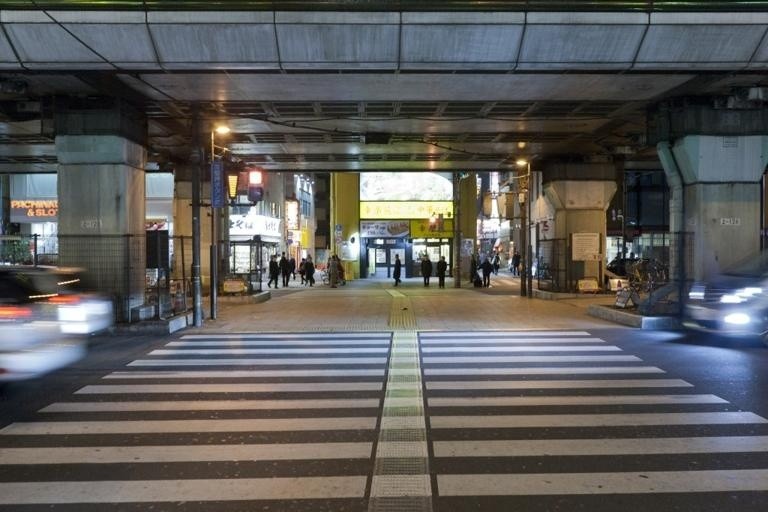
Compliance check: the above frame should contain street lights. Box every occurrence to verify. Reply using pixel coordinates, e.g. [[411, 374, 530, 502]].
[[516, 158, 532, 298], [211, 126, 229, 319]]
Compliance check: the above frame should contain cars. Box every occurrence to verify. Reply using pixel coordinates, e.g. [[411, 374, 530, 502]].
[[678, 251, 768, 345], [0, 265, 115, 382]]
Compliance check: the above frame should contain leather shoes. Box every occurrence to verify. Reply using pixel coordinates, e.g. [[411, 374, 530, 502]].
[[267, 280, 315, 289], [393, 280, 445, 289]]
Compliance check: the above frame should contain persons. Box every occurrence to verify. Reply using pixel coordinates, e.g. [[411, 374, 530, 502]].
[[421, 255, 432, 285], [615, 252, 635, 259], [327, 255, 345, 288], [393, 254, 401, 287], [471, 250, 501, 287], [268, 251, 315, 288], [511, 251, 521, 276], [437, 256, 447, 288]]
